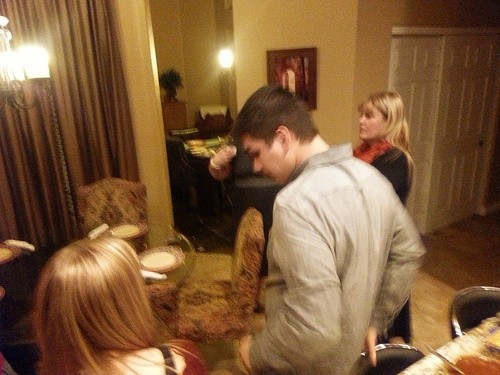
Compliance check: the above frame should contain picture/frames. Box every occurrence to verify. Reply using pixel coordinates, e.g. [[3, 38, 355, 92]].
[[266, 47, 319, 109]]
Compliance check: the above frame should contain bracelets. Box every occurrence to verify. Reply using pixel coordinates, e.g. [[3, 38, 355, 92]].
[[211, 158, 221, 170]]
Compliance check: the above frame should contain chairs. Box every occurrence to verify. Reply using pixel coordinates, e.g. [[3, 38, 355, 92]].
[[77, 180, 149, 255], [151, 210, 266, 344]]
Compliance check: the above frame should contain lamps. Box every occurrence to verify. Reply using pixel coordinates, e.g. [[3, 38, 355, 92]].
[[0, 14, 51, 112]]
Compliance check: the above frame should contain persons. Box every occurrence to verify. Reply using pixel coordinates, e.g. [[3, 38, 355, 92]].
[[208, 132, 283, 312], [34, 237, 208, 375], [352, 90, 415, 346], [232, 84, 427, 375]]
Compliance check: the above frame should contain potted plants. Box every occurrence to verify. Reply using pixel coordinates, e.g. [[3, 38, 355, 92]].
[[160, 67, 188, 132]]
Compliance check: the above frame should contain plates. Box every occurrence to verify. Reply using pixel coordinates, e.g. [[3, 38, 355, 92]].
[[109, 224, 140, 239], [138, 248, 186, 272]]
